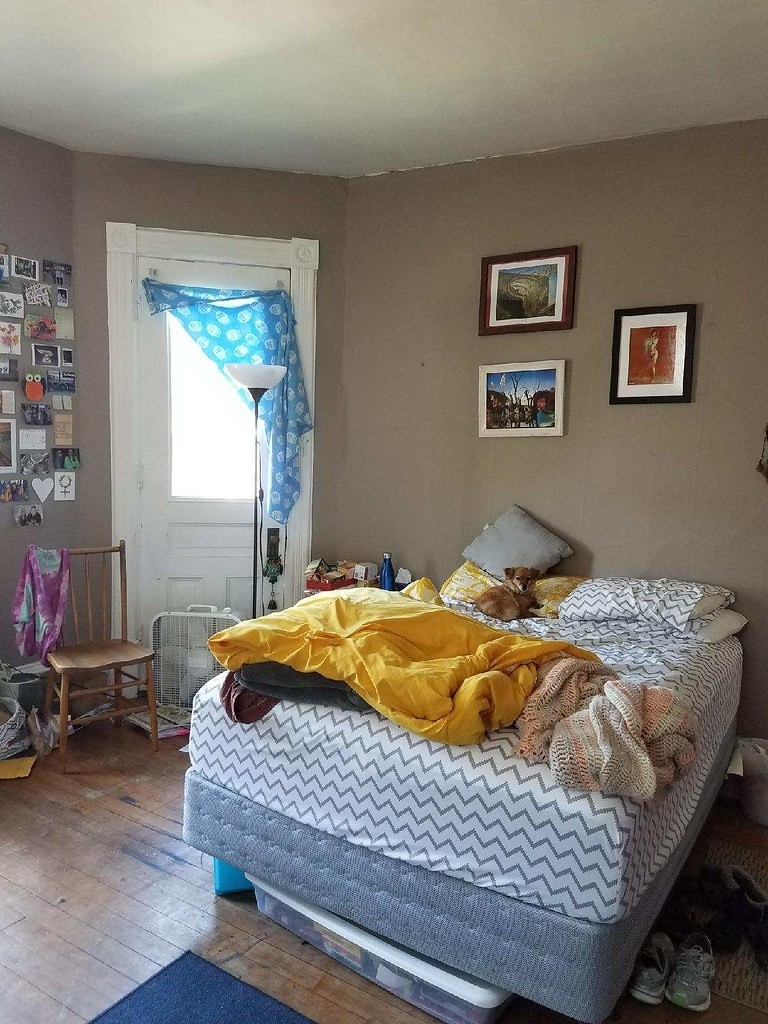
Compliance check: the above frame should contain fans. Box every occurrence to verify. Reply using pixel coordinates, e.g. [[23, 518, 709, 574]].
[[149, 604, 250, 711]]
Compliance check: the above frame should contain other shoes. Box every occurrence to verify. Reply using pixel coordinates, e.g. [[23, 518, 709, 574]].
[[746, 904, 768, 970], [668, 890, 746, 954]]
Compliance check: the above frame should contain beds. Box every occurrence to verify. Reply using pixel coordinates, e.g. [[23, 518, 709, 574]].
[[183, 615, 744, 1024]]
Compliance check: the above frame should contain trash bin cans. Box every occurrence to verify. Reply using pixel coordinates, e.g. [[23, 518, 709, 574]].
[[737, 736, 767, 824]]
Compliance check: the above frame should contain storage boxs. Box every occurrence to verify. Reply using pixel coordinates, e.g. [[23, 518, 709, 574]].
[[357, 580, 378, 588], [338, 560, 357, 580], [323, 571, 346, 583], [305, 578, 357, 591], [68, 671, 108, 720], [244, 872, 518, 1024], [354, 562, 379, 580], [304, 557, 332, 578]]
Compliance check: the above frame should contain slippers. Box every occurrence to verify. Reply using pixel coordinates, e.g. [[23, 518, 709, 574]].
[[687, 862, 768, 914]]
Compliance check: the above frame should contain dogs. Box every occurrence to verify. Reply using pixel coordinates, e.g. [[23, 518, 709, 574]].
[[476, 566, 544, 623]]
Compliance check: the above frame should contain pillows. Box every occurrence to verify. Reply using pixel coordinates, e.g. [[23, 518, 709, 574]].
[[439, 559, 590, 618], [558, 576, 736, 633], [462, 502, 575, 583], [672, 608, 750, 643]]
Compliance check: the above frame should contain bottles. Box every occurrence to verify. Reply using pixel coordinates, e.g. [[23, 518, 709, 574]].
[[380, 552, 395, 591]]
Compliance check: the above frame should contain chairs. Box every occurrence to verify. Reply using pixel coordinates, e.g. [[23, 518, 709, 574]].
[[28, 539, 158, 774]]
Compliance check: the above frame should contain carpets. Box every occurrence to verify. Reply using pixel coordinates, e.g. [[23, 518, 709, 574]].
[[639, 838, 768, 1014], [88, 949, 319, 1024]]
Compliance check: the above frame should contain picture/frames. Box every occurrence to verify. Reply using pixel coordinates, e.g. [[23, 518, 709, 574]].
[[0, 419, 17, 474], [608, 304, 696, 405], [478, 359, 567, 437], [478, 245, 578, 335]]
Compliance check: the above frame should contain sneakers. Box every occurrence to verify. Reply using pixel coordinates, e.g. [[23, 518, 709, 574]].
[[629, 932, 675, 1005], [665, 933, 715, 1012]]
